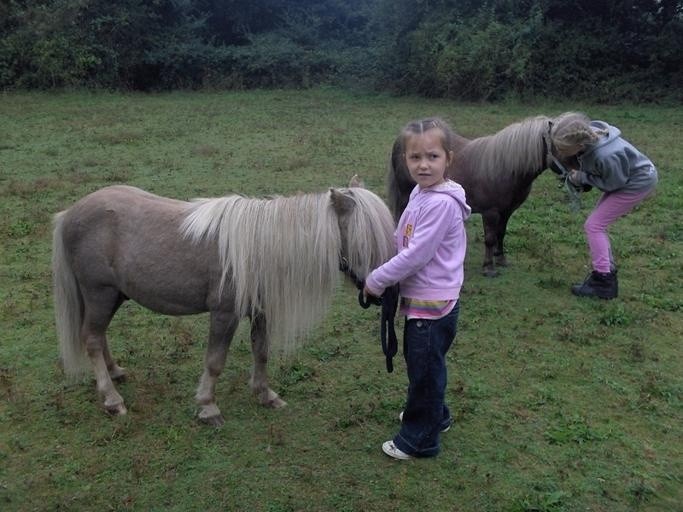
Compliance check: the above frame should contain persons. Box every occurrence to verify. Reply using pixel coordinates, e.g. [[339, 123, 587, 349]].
[[548, 110, 658, 301], [363, 117, 471, 460]]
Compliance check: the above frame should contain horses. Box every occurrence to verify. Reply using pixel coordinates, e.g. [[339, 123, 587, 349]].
[[48, 173, 401, 429], [386, 112, 596, 280]]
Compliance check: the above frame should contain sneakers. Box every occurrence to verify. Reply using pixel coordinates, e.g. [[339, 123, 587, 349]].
[[399, 410, 452, 434], [381, 439, 415, 460]]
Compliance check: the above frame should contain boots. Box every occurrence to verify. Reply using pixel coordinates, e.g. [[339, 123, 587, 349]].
[[570, 266, 620, 299]]
